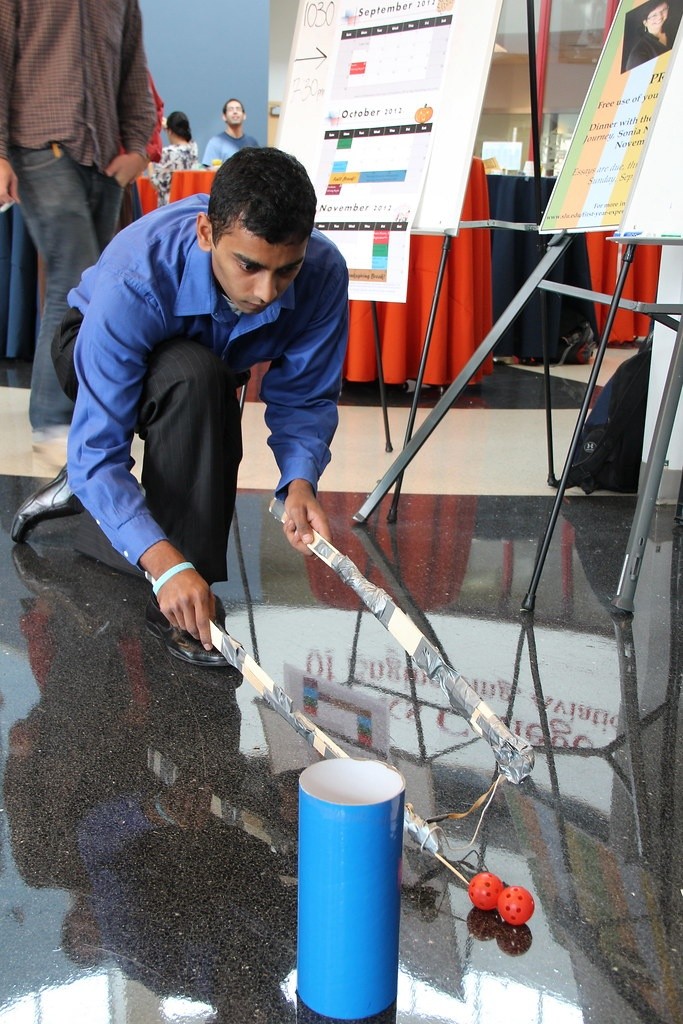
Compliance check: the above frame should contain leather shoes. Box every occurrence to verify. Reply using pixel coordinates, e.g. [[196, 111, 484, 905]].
[[144, 589, 232, 667], [10, 454, 135, 543]]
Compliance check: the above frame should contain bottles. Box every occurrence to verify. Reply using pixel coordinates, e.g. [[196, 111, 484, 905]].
[[212, 159, 223, 171]]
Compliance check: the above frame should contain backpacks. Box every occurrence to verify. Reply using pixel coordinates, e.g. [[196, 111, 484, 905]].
[[563, 348, 652, 495]]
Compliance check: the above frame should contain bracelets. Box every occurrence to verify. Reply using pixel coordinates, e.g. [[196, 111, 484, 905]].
[[143, 561, 196, 596]]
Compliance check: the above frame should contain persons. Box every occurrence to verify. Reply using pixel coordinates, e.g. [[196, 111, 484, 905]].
[[626, 0, 675, 71], [0, 0, 157, 468], [10, 146, 349, 668], [200, 98, 260, 169], [146, 111, 198, 208]]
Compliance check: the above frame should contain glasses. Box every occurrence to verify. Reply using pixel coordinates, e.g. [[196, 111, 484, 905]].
[[646, 6, 669, 20]]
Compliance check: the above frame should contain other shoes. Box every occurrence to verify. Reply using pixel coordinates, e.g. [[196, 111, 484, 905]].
[[32, 423, 70, 467]]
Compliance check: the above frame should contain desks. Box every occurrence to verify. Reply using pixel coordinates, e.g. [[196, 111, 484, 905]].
[[170, 154, 662, 398]]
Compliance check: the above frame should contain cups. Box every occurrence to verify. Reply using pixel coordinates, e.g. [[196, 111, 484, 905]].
[[297, 759, 406, 1020]]
